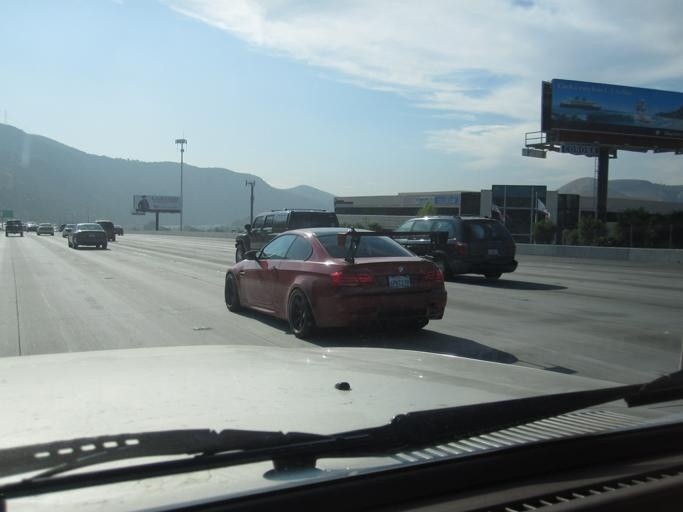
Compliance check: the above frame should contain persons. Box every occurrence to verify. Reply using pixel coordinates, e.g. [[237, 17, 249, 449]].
[[138, 196, 149, 210]]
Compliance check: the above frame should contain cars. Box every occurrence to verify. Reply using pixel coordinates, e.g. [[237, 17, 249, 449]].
[[225, 225, 446, 336], [0, 220, 124, 249]]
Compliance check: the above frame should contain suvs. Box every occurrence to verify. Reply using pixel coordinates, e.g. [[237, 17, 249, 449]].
[[230, 208, 339, 266], [389, 215, 519, 282]]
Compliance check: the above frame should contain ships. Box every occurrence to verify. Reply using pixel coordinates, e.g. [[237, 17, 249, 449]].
[[559, 96, 601, 110]]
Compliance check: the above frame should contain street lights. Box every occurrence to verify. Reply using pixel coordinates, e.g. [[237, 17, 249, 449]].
[[243, 178, 255, 226], [175, 137, 187, 230]]
